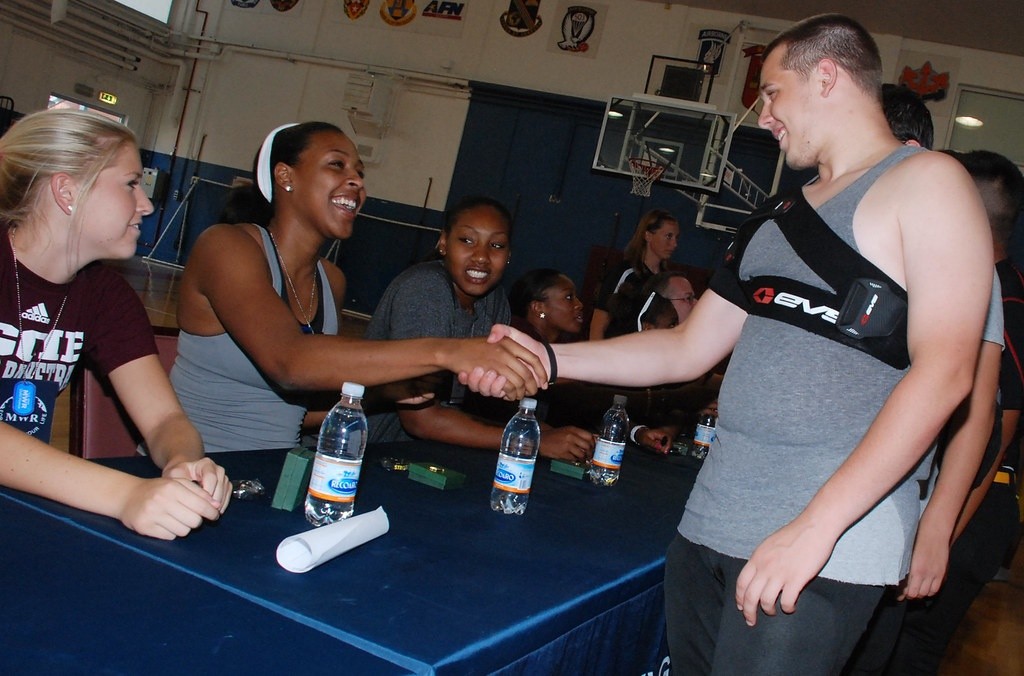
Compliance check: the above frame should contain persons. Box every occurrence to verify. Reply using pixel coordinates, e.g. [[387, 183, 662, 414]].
[[587, 283, 718, 421], [299, 197, 595, 462], [460, 13, 995, 676], [0, 109, 233, 540], [590, 207, 679, 341], [473, 267, 671, 453], [134, 121, 548, 458], [840, 82, 1024, 676], [635, 273, 724, 409]]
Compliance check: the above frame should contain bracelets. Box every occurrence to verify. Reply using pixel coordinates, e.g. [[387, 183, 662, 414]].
[[631, 425, 648, 446]]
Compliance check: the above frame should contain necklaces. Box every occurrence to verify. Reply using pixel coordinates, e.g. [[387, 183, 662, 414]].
[[10, 226, 68, 416], [268, 230, 318, 334]]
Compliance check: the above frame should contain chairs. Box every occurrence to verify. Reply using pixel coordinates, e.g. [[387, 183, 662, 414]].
[[67, 334, 177, 459]]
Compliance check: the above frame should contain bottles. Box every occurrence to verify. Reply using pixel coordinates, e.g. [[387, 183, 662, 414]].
[[490, 397, 541, 515], [590, 393, 629, 486], [686, 405, 718, 460], [303, 381, 367, 528]]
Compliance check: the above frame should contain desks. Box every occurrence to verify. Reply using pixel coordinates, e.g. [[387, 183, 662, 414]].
[[0, 431, 703, 676]]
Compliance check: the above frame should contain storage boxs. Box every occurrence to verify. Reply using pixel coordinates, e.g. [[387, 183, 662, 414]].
[[548, 458, 588, 482], [405, 457, 467, 492], [271, 446, 316, 510]]
